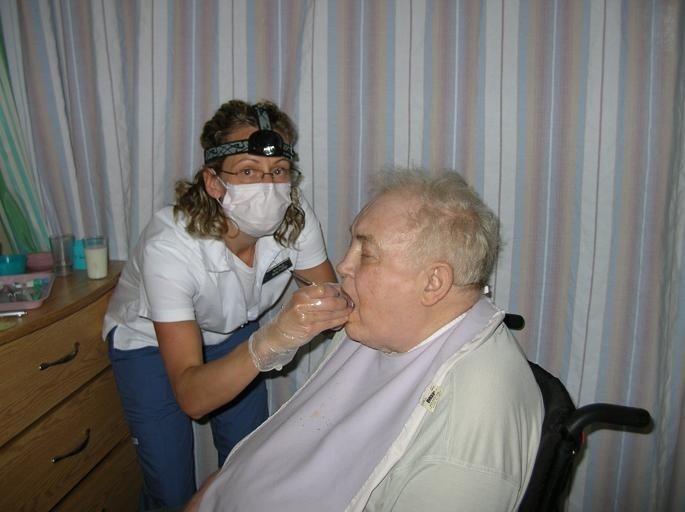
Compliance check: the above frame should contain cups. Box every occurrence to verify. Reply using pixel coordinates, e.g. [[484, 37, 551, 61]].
[[48, 234, 109, 280]]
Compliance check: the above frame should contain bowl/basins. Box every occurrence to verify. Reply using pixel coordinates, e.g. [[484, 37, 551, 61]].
[[0, 253, 53, 276]]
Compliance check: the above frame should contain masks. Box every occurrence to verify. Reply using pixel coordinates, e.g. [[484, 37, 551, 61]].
[[210, 171, 292, 238]]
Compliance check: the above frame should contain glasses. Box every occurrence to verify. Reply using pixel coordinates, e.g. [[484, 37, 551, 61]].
[[213, 167, 290, 184]]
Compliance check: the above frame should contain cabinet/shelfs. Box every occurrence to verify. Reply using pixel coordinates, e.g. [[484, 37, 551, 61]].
[[0, 262, 142, 512]]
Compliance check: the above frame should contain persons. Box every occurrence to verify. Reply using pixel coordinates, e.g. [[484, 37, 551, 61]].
[[182, 159, 546, 512], [102, 99, 353, 512]]
